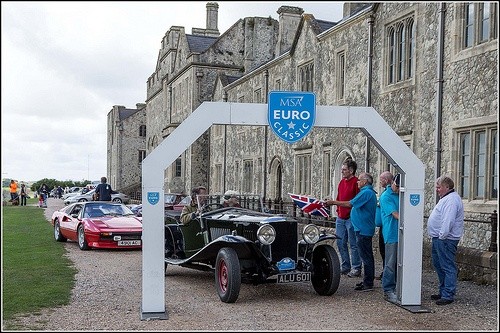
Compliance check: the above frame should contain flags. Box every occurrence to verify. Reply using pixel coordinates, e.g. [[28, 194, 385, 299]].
[[288, 192, 330, 220]]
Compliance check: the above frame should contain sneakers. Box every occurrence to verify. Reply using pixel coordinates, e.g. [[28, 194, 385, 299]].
[[341, 269, 351, 275], [383, 291, 397, 303], [431, 295, 441, 299], [354, 282, 374, 292], [347, 269, 362, 278], [436, 298, 454, 305]]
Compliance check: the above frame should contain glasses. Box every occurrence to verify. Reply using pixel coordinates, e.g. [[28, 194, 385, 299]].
[[357, 179, 364, 181]]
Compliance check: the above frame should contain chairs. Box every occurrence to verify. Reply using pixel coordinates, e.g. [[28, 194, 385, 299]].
[[93, 209, 105, 217]]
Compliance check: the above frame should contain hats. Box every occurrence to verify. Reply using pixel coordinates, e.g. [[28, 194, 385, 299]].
[[392, 174, 400, 188]]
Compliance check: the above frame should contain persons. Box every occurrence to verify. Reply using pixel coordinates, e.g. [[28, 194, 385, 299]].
[[336, 160, 362, 278], [9, 179, 96, 205], [426, 175, 464, 306], [95, 176, 112, 201], [374, 171, 400, 299], [327, 173, 377, 292], [221, 189, 242, 208], [180, 185, 213, 224]]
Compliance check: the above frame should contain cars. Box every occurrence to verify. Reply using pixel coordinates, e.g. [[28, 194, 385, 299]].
[[165, 194, 341, 304], [127, 205, 142, 222], [52, 201, 142, 250], [49, 184, 130, 204]]
